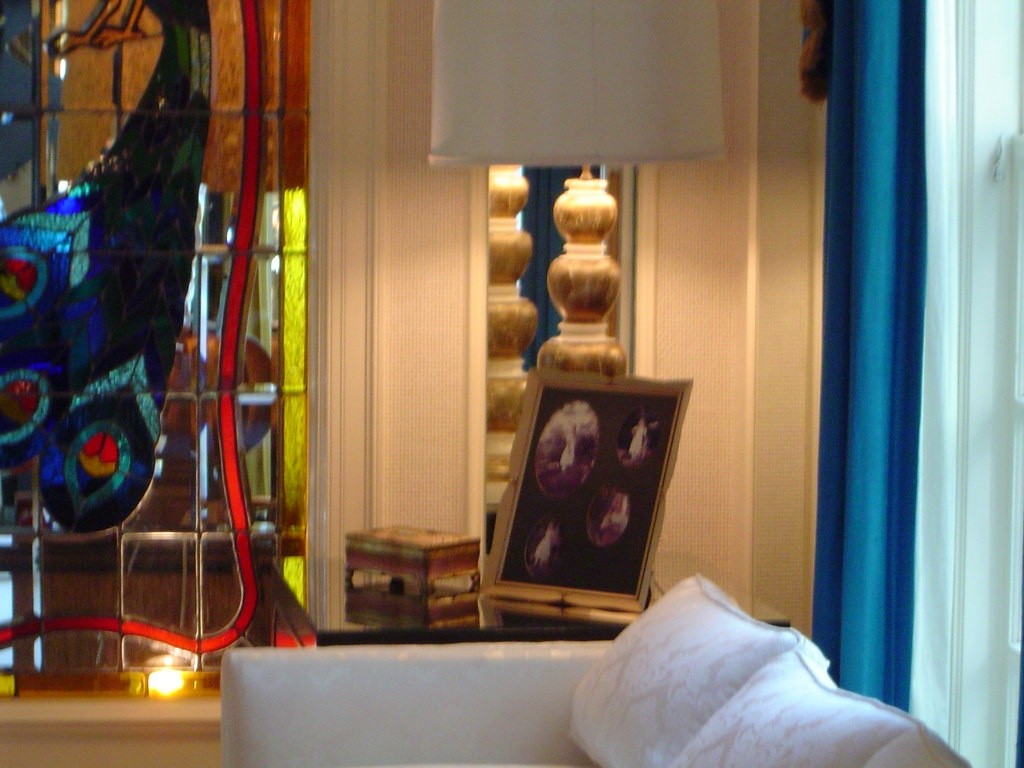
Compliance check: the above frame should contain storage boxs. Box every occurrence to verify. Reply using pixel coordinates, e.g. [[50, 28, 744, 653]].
[[344, 525, 482, 600]]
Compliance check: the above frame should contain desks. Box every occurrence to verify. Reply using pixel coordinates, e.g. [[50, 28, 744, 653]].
[[272, 555, 791, 647]]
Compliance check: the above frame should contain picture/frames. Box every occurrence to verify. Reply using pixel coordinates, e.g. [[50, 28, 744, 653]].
[[480, 367, 695, 623]]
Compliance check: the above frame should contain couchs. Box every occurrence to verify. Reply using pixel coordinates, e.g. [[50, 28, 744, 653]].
[[220, 573, 611, 768]]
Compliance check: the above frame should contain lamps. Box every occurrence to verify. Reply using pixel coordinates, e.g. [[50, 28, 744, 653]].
[[426, 1, 727, 376]]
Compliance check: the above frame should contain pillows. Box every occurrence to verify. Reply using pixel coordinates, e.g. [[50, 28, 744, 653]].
[[569, 572, 830, 768], [670, 642, 973, 768]]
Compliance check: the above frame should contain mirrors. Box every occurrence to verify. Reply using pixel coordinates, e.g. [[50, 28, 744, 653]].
[[490, 165, 635, 518]]
